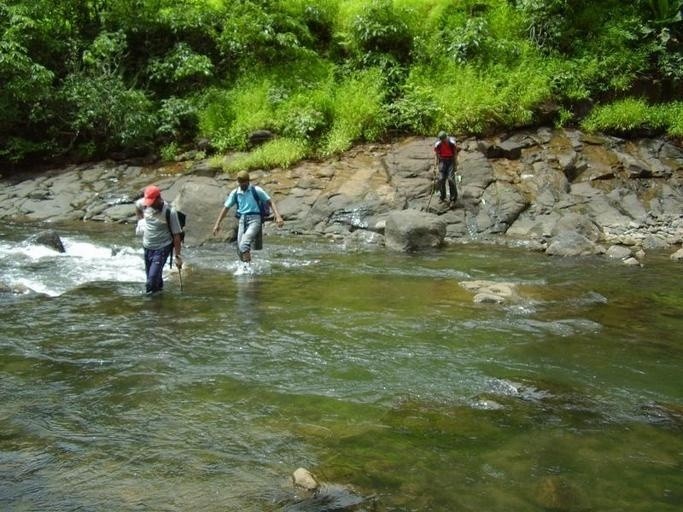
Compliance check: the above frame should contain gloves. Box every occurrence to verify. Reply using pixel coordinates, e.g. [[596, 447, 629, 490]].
[[175, 255, 183, 271]]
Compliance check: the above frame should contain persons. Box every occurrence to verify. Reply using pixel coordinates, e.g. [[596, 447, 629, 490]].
[[133, 185, 184, 294], [212, 170, 283, 266], [430, 130, 460, 208]]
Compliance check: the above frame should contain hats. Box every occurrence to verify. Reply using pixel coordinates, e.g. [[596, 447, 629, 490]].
[[438, 131, 448, 140], [144, 185, 160, 206], [235, 170, 250, 181]]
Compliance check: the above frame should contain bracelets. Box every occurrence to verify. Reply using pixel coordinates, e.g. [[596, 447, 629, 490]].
[[175, 255, 181, 259]]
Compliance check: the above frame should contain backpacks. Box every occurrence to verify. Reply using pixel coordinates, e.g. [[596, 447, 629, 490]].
[[166, 202, 187, 243], [234, 185, 269, 224]]
